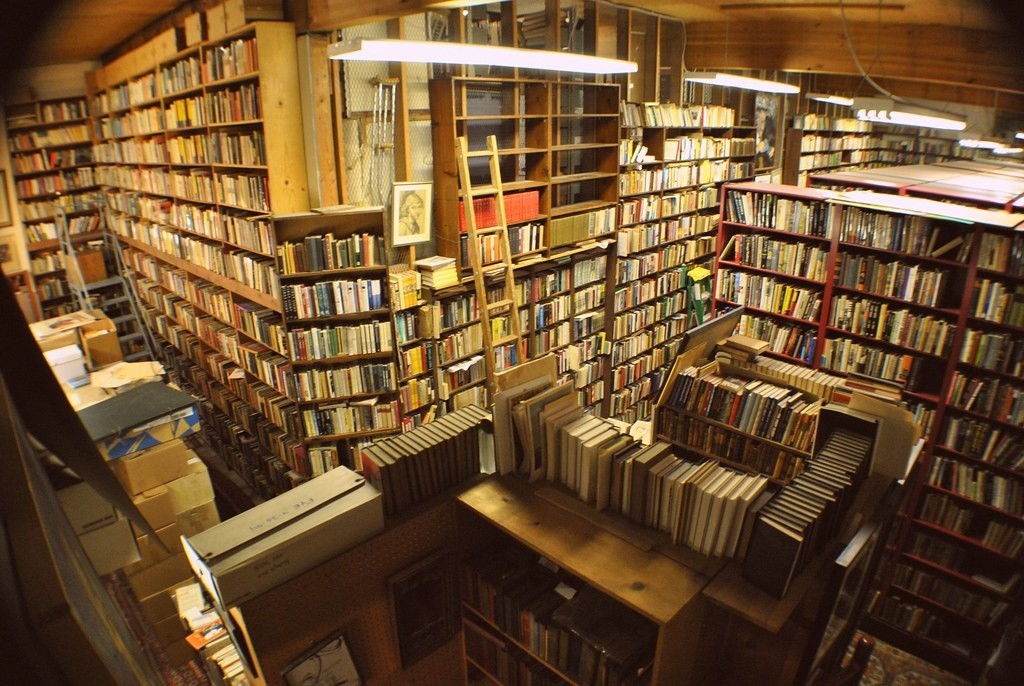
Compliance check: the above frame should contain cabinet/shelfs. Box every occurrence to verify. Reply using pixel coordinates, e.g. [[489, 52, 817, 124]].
[[0, 21, 1024, 521], [711, 180, 1024, 686], [456, 358, 926, 686]]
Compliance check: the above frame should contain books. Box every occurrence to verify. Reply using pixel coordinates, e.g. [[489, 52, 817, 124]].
[[0, 38, 1024, 686]]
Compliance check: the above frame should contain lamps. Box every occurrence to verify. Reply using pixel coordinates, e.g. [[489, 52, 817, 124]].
[[683, 9, 801, 94], [327, 6, 638, 74], [850, 0, 968, 130], [805, 53, 881, 106]]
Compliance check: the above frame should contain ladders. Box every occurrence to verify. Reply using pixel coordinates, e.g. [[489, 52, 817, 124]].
[[52, 195, 156, 362], [453, 132, 527, 412]]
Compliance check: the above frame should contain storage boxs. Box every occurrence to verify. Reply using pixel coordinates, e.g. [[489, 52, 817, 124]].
[[29, 309, 221, 669], [180, 465, 386, 612], [66, 250, 106, 285], [83, 0, 285, 93]]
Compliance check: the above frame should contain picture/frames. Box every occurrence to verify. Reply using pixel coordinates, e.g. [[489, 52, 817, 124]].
[[388, 548, 462, 675], [1, 378, 168, 686], [0, 169, 13, 228], [391, 181, 434, 246], [279, 626, 367, 686]]
